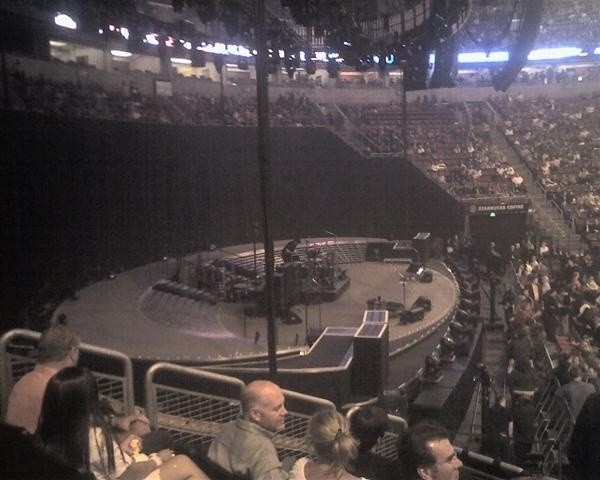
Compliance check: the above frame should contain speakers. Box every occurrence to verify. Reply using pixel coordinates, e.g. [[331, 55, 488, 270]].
[[407, 264, 425, 273], [400, 306, 425, 322], [414, 232, 433, 262], [412, 295, 431, 311], [416, 267, 433, 282]]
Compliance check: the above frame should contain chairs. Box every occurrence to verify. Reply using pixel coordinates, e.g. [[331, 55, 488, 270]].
[[0, 422, 237, 480]]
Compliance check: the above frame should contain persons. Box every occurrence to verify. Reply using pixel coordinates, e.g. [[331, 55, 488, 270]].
[[332, 131, 600, 242], [0, 56, 599, 132], [280, 237, 302, 264], [1, 322, 466, 479], [429, 243, 600, 480]]
